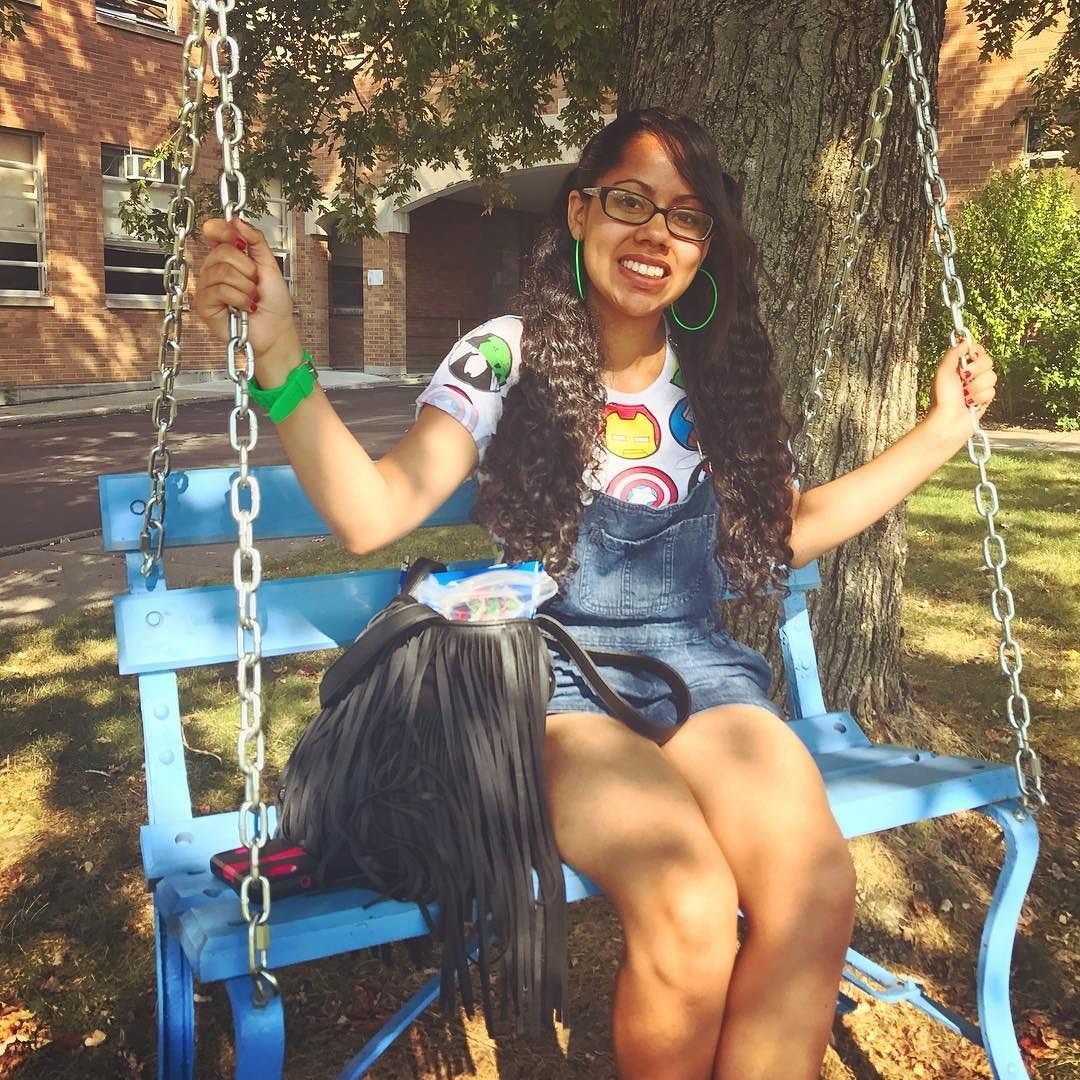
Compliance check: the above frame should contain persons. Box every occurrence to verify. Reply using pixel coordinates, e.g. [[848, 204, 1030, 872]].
[[192, 107, 998, 1080]]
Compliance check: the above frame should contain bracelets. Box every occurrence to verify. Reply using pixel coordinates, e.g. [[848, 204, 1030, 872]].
[[247, 347, 319, 424]]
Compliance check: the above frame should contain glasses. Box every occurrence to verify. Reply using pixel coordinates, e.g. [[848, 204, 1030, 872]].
[[582, 187, 719, 242]]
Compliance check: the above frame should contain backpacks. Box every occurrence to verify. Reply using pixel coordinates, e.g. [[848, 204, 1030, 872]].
[[273, 557, 692, 1039]]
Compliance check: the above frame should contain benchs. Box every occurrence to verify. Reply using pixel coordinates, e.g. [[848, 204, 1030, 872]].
[[101, 444, 1043, 1080]]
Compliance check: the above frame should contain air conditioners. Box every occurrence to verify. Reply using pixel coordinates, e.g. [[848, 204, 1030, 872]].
[[123, 154, 164, 183]]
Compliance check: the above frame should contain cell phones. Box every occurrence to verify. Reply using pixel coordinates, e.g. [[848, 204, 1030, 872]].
[[210, 839, 321, 905]]
